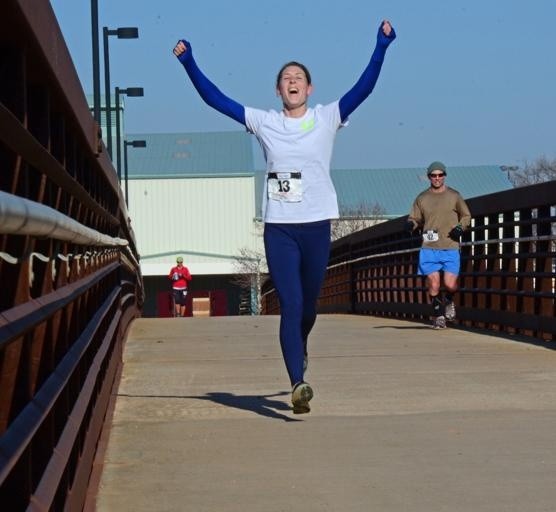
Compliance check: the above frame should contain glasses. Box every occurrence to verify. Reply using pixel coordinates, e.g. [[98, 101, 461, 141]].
[[430, 173, 445, 177]]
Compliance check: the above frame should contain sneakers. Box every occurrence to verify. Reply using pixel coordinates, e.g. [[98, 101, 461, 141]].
[[444, 293, 457, 320], [292, 354, 313, 414], [432, 314, 446, 329]]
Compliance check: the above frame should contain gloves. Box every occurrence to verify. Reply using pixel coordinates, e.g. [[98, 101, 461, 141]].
[[448, 226, 463, 242]]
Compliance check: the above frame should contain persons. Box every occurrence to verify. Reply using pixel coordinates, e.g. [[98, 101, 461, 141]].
[[172, 17, 398, 413], [168, 257, 192, 317], [404, 162, 472, 328]]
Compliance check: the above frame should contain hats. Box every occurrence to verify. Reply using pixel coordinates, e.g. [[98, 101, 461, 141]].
[[427, 162, 446, 175]]
